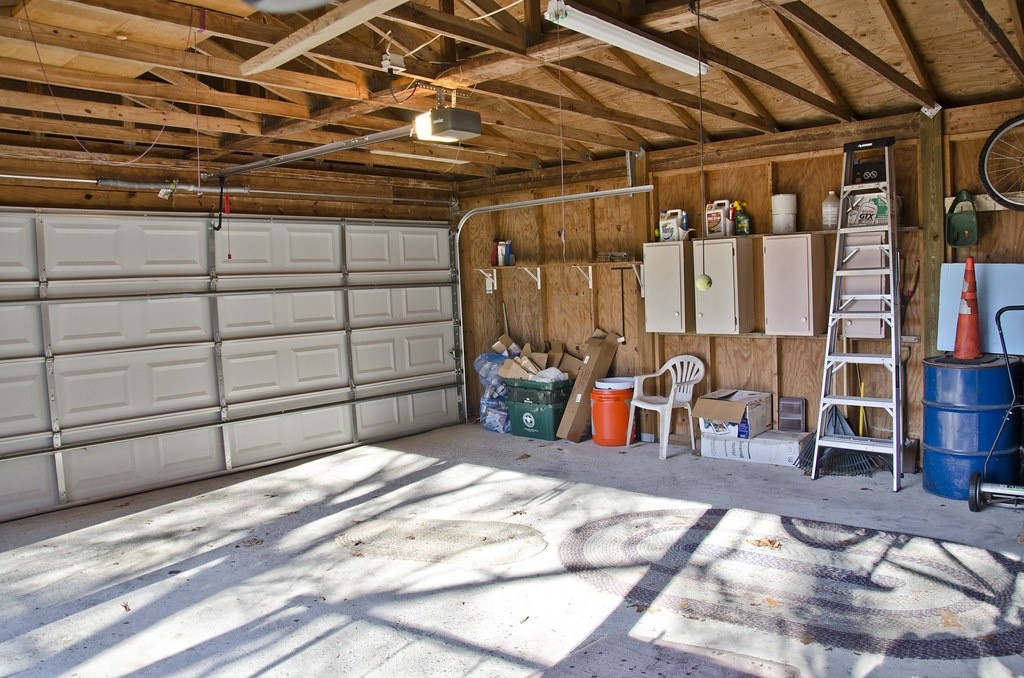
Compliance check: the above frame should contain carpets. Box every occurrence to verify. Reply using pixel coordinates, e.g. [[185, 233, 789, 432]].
[[0, 446, 1024, 678]]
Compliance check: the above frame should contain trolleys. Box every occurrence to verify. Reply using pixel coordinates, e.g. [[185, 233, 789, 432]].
[[968, 304, 1024, 512]]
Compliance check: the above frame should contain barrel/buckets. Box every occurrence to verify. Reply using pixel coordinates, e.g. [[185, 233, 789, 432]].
[[705, 199, 730, 238], [658, 209, 683, 241], [921, 355, 1021, 500]]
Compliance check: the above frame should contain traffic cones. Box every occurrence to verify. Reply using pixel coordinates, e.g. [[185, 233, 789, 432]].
[[934, 255, 1000, 365]]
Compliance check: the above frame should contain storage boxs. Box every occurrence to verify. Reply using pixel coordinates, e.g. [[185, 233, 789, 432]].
[[688, 386, 816, 469], [556, 328, 623, 443], [847, 192, 906, 227], [503, 376, 576, 443]]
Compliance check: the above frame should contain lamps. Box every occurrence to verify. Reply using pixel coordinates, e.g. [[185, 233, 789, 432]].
[[546, 0, 709, 79], [198, 107, 481, 183]]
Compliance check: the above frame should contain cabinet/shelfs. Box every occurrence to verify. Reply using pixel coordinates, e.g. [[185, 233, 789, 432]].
[[643, 232, 828, 337]]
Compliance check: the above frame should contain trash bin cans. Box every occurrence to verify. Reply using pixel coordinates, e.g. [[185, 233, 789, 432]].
[[503, 381, 576, 442]]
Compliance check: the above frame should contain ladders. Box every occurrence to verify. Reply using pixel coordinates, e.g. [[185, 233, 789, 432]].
[[810, 135, 905, 492]]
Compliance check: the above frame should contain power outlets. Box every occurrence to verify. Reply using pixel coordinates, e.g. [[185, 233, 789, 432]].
[[382, 53, 406, 75]]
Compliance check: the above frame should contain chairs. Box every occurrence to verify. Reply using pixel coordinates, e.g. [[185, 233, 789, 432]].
[[622, 353, 706, 460]]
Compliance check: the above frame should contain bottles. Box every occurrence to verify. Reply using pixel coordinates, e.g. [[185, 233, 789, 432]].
[[821, 191, 840, 230], [590, 387, 636, 446], [491, 239, 512, 266]]
